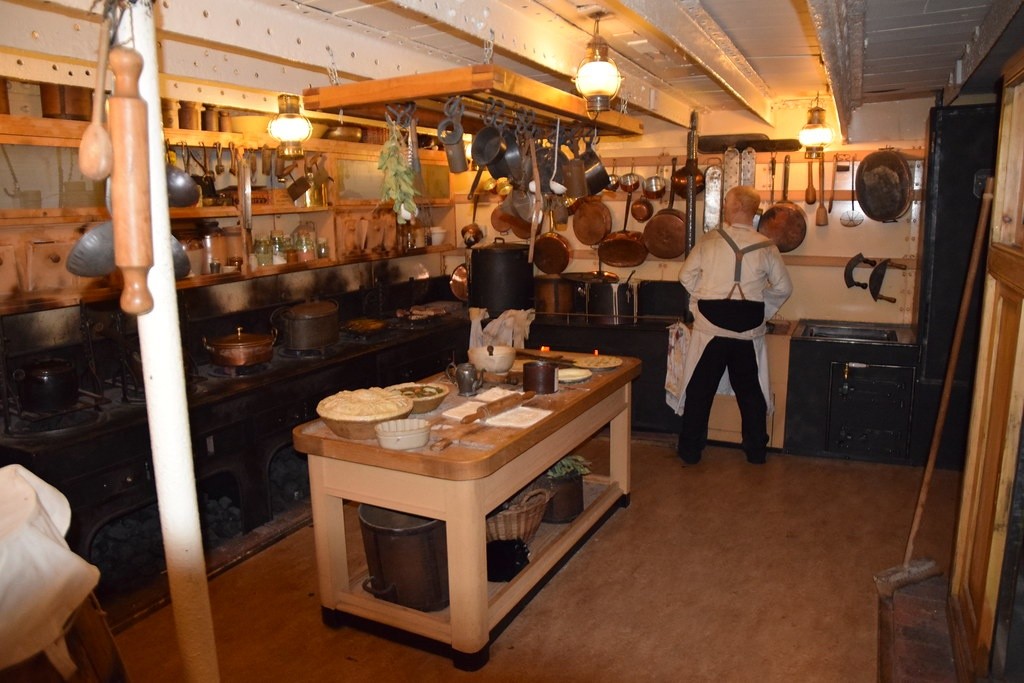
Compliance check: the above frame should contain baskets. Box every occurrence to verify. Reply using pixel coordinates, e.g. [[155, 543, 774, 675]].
[[486, 489, 555, 545]]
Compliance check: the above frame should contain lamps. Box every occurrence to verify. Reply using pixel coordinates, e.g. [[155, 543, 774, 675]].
[[799, 91, 834, 159], [268, 94, 312, 160], [571, 16, 626, 121]]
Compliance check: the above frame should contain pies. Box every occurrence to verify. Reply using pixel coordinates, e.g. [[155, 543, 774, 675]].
[[571, 356, 622, 368]]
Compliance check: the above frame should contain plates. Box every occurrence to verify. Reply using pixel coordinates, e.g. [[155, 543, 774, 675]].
[[573, 356, 623, 371], [558, 368, 592, 383]]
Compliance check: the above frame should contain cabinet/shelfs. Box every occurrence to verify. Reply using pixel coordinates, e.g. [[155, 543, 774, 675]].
[[0, 78, 458, 317]]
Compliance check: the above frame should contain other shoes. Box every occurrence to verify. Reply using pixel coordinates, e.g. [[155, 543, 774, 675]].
[[676, 442, 701, 464], [747, 448, 768, 464]]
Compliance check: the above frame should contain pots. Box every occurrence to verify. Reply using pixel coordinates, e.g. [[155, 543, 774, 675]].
[[279, 298, 340, 351], [448, 125, 915, 327], [65, 159, 200, 280], [13, 355, 82, 411], [121, 338, 195, 393], [201, 327, 278, 368]]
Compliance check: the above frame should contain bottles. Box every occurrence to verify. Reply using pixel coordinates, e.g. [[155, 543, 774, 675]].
[[162, 97, 232, 133], [173, 173, 331, 283]]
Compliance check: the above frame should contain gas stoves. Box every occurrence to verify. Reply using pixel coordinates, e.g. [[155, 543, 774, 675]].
[[0, 307, 468, 459]]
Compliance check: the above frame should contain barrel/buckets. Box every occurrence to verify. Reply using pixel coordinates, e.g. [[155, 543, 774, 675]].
[[538, 473, 584, 524], [358, 502, 449, 612]]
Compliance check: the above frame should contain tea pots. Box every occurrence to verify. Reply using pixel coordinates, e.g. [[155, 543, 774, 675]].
[[445, 362, 487, 396]]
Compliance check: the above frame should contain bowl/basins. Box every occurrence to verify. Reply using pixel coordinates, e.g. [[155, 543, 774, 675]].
[[318, 391, 450, 450], [39, 81, 94, 121], [430, 227, 446, 246], [467, 346, 516, 376], [232, 115, 362, 142]]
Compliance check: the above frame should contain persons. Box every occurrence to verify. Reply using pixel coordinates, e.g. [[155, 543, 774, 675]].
[[678, 185, 794, 465]]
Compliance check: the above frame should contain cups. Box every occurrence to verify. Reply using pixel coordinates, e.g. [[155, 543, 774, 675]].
[[19, 190, 42, 209], [523, 362, 559, 395]]
